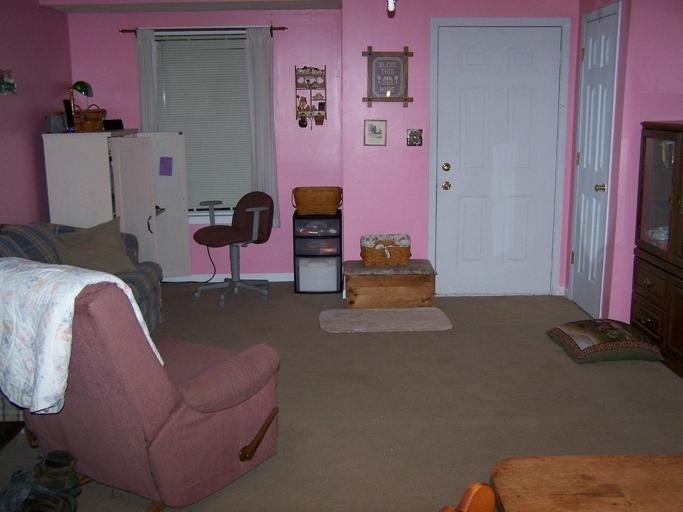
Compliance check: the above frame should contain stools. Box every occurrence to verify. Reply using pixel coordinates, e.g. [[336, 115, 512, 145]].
[[342, 258, 438, 309]]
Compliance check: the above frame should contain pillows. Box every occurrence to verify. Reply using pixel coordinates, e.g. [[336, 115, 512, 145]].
[[50, 216, 136, 274], [545, 317, 666, 364]]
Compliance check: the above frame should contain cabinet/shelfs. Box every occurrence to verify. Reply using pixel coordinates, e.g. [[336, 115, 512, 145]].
[[39, 128, 192, 279], [629, 118, 683, 377], [292, 208, 341, 295], [294, 65, 326, 120]]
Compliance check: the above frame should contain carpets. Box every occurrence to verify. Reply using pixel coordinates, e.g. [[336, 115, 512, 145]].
[[318, 308, 453, 334]]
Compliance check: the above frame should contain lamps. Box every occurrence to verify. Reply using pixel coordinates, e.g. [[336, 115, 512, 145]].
[[68, 80, 93, 116]]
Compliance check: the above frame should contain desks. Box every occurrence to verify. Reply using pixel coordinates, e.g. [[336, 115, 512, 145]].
[[489, 454, 683, 511]]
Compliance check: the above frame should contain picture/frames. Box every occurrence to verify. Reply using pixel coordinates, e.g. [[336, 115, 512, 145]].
[[363, 120, 386, 147]]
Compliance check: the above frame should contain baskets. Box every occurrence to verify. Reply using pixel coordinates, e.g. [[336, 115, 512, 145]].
[[73, 104, 107, 132], [360, 233, 407, 266], [291, 186, 343, 216]]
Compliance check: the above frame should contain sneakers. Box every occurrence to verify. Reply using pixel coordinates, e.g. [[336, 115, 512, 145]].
[[0, 449, 81, 512]]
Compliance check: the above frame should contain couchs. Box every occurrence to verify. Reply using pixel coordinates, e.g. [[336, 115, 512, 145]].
[[0, 220, 162, 425]]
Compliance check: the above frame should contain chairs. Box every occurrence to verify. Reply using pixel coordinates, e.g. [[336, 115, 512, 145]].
[[0, 257, 280, 512], [192, 191, 274, 307]]
